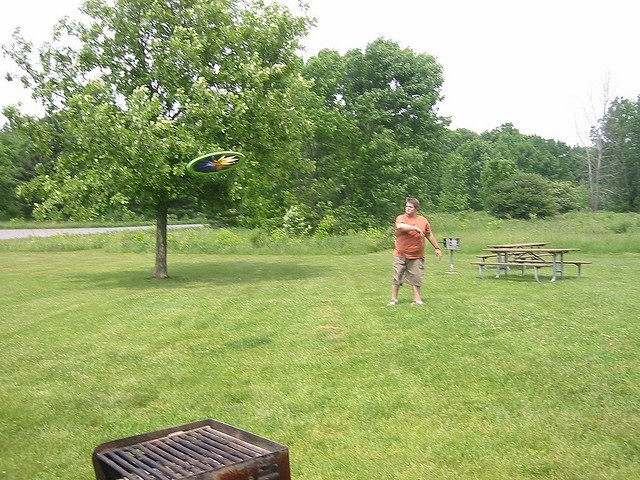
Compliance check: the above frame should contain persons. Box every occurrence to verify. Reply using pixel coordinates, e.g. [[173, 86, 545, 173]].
[[389, 197, 442, 305]]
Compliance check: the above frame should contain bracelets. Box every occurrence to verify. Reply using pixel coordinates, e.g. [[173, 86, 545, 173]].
[[435, 248, 439, 249]]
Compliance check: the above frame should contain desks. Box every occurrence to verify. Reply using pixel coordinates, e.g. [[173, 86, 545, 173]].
[[482, 248, 582, 276], [487, 242, 550, 270]]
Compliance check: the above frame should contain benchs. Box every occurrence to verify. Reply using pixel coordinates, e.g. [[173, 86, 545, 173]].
[[516, 260, 592, 280], [476, 255, 523, 265], [515, 253, 557, 269], [470, 262, 553, 283]]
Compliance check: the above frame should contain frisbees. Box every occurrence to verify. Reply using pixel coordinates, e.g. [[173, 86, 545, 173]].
[[187, 151, 245, 175]]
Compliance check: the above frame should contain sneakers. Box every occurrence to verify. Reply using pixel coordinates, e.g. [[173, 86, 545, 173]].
[[387, 299, 398, 305], [411, 300, 424, 305]]
